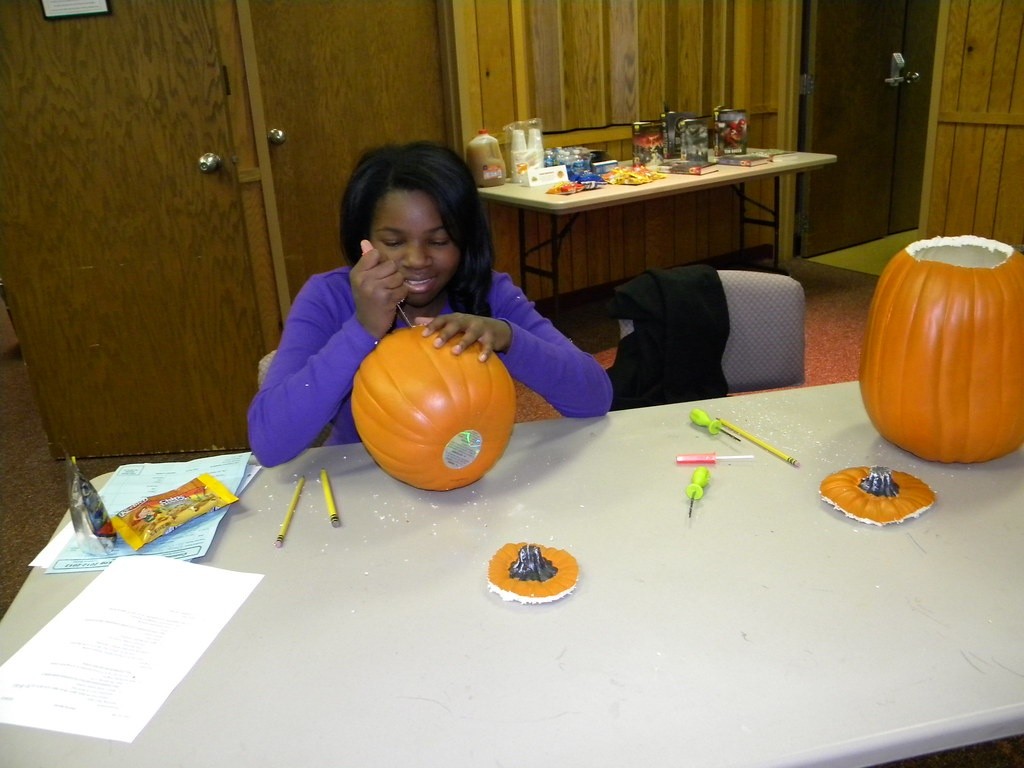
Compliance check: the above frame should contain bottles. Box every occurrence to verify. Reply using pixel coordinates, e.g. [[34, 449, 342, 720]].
[[544, 146, 591, 174], [466, 129, 506, 188]]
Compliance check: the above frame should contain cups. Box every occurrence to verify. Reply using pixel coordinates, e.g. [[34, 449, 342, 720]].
[[510, 130, 527, 183], [527, 129, 544, 181]]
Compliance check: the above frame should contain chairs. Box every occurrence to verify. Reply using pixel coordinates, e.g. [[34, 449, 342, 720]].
[[605, 264, 804, 417]]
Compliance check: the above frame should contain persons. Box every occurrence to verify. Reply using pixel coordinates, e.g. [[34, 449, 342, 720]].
[[246, 140, 613, 467]]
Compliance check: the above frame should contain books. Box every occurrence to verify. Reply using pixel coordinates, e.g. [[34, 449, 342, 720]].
[[714, 106, 747, 156], [657, 159, 720, 176], [715, 153, 770, 167], [633, 122, 664, 166], [661, 112, 708, 162]]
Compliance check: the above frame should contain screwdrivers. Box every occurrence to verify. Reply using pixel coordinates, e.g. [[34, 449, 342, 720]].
[[686, 465, 711, 519], [690, 407, 743, 443]]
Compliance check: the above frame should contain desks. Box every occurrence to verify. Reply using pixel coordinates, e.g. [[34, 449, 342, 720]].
[[476, 146, 839, 323], [0, 380, 1024, 768]]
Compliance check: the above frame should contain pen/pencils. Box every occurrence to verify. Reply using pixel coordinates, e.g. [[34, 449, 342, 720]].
[[714, 415, 802, 468], [319, 468, 342, 529], [274, 474, 306, 549]]
